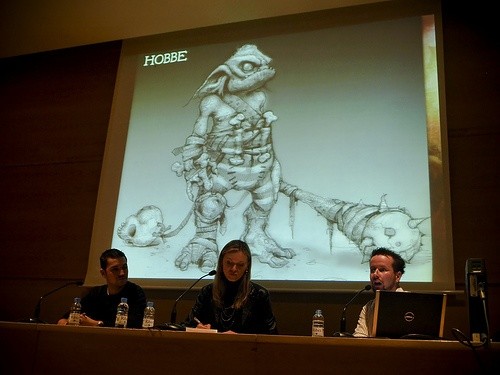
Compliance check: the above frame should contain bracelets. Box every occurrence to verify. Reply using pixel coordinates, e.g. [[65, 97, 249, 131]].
[[98, 321, 103, 326]]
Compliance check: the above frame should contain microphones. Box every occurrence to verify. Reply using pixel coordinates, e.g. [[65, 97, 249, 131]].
[[478, 288, 490, 343], [332, 285, 372, 337], [156, 269, 217, 331], [25, 280, 85, 324]]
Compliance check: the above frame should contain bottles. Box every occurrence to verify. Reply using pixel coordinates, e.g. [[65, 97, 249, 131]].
[[142, 301, 155, 329], [115, 297, 128, 328], [312, 310, 324, 337], [68, 297, 81, 326]]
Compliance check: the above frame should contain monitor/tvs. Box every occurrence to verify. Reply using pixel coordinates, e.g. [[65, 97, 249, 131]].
[[371, 289, 447, 340]]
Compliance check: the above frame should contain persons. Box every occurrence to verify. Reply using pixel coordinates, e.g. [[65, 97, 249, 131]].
[[351, 248, 408, 338], [179, 239, 275, 336], [58, 249, 147, 329]]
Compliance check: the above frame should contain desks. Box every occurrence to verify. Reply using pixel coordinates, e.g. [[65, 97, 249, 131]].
[[0, 321, 500, 375]]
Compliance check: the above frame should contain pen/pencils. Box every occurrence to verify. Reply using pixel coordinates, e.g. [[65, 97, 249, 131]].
[[194, 317, 201, 323]]
